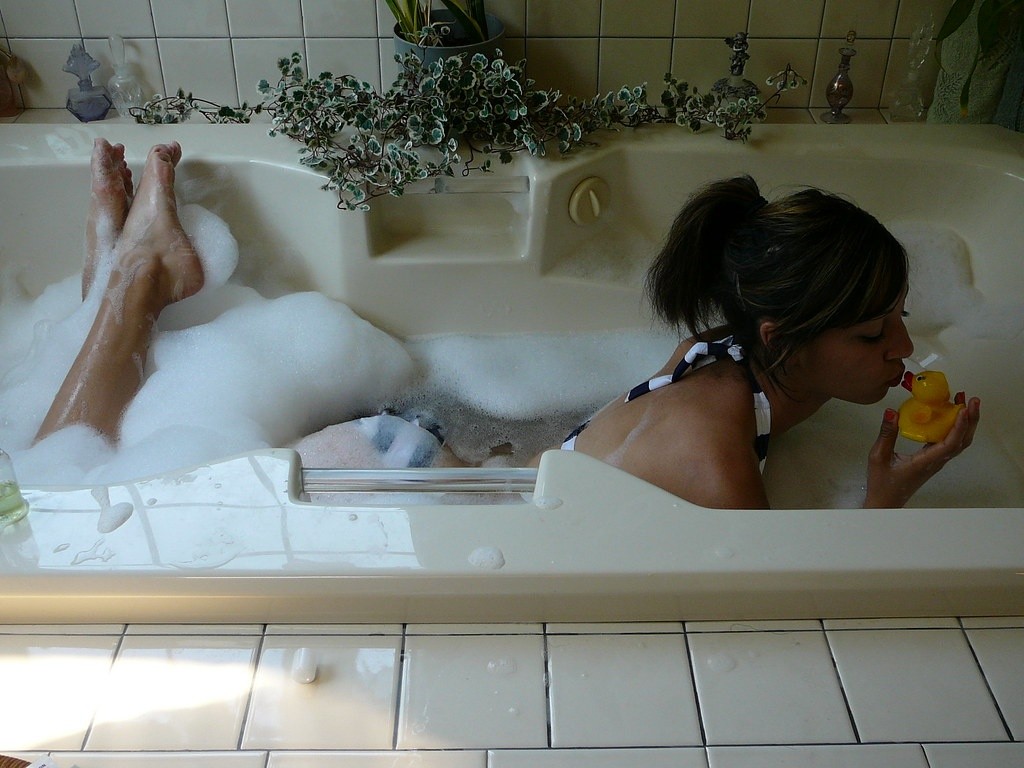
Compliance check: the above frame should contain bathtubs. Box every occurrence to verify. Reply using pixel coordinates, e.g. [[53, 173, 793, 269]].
[[0, 122, 1023, 622]]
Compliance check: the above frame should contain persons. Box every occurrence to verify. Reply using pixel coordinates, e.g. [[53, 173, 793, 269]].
[[31, 137, 979, 509]]
